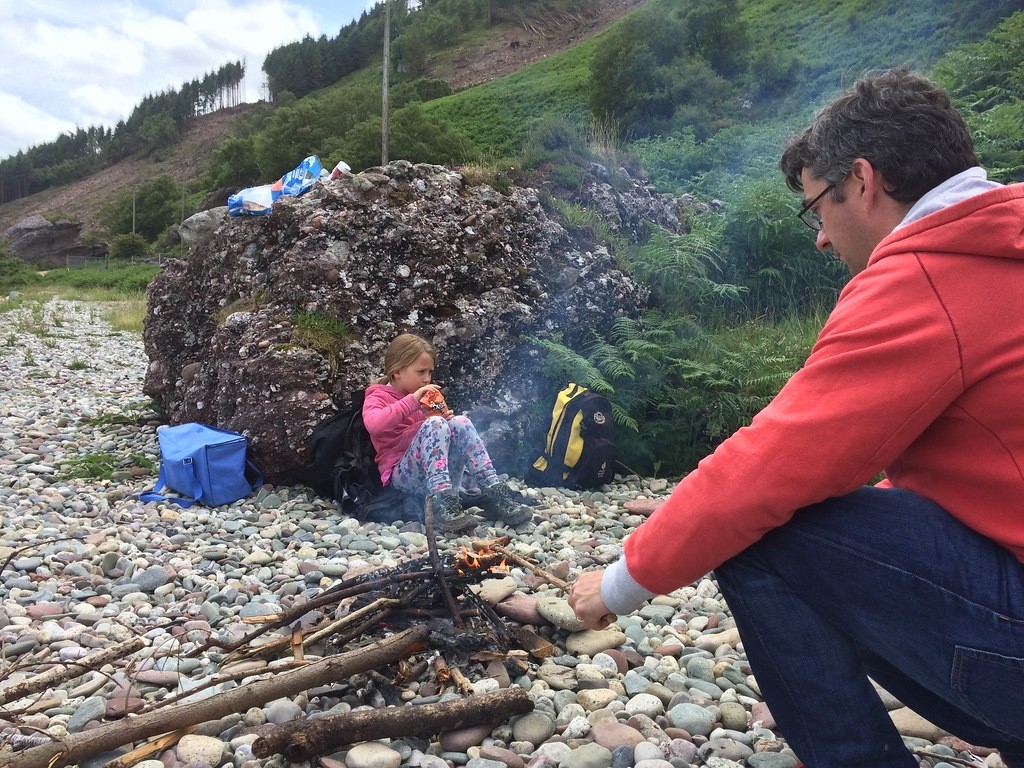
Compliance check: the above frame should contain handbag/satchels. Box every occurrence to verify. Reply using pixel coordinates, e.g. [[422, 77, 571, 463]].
[[138, 422, 263, 509], [228, 156, 322, 217]]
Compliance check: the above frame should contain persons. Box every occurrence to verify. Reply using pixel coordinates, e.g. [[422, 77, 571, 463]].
[[361, 333, 534, 531], [567, 68, 1023, 768]]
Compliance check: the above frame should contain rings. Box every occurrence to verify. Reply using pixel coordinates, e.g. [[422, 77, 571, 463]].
[[577, 617, 584, 623]]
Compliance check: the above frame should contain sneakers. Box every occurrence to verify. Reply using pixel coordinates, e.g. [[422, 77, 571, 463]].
[[432, 490, 478, 531], [483, 482, 533, 526]]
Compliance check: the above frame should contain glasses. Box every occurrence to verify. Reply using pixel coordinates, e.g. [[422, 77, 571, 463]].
[[797, 175, 843, 231]]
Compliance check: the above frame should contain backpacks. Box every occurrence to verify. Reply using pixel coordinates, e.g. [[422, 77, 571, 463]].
[[524, 381, 617, 489]]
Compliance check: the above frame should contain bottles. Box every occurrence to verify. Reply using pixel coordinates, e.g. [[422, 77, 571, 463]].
[[329, 161, 351, 182]]
